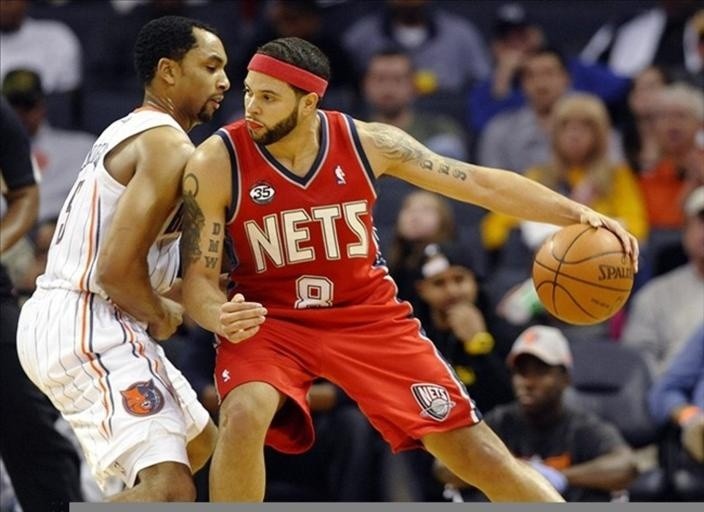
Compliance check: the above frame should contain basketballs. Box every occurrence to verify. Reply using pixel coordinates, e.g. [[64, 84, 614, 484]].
[[533, 225, 635, 324]]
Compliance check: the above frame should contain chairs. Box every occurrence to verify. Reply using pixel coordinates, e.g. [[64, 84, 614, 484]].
[[376, 174, 638, 276], [561, 334, 638, 415]]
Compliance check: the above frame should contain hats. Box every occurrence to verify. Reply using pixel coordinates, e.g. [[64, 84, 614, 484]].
[[502, 324, 576, 376], [408, 241, 487, 286]]
